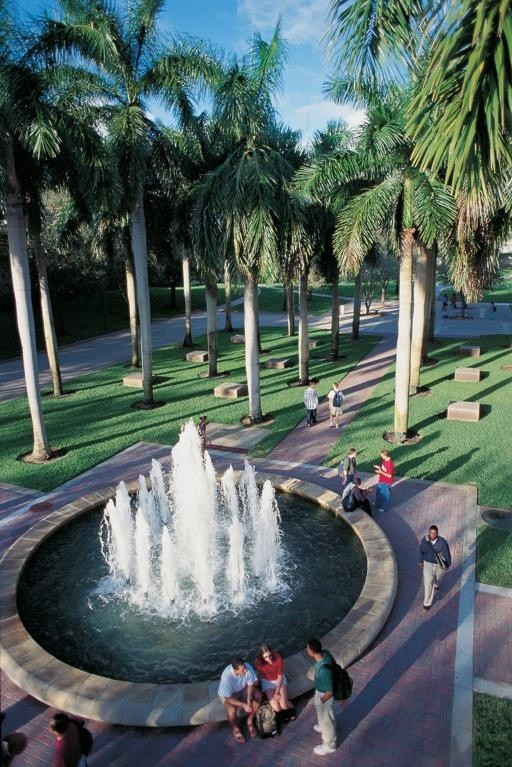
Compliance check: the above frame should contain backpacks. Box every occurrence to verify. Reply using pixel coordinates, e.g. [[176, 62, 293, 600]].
[[255, 700, 281, 739], [332, 389, 342, 407], [341, 486, 358, 512], [70, 719, 94, 757], [319, 651, 354, 701], [338, 458, 355, 479]]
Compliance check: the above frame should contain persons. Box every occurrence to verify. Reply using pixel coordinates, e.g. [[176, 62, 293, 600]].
[[417, 524, 452, 611], [302, 379, 319, 427], [340, 447, 357, 490], [449, 291, 458, 310], [372, 449, 394, 512], [217, 654, 263, 743], [196, 415, 211, 455], [305, 636, 339, 755], [339, 477, 374, 519], [253, 640, 297, 722], [46, 710, 88, 766], [440, 293, 448, 312], [325, 381, 344, 429], [488, 301, 497, 320]]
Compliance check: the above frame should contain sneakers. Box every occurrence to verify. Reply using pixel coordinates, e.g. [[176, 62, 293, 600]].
[[330, 422, 341, 429], [231, 731, 248, 746], [376, 507, 385, 514], [313, 744, 337, 756], [435, 586, 441, 593], [247, 724, 258, 738], [313, 723, 322, 734], [282, 708, 297, 724], [422, 604, 433, 612], [306, 420, 318, 427]]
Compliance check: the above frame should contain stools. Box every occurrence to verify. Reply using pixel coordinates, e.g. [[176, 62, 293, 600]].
[[455, 307, 477, 312], [454, 368, 479, 382], [447, 401, 480, 422], [122, 333, 320, 399], [459, 345, 480, 357]]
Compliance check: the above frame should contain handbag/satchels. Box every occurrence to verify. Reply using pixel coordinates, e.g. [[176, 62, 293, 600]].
[[424, 535, 448, 571]]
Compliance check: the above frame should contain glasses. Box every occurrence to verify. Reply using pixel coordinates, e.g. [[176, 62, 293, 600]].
[[263, 654, 273, 660]]
